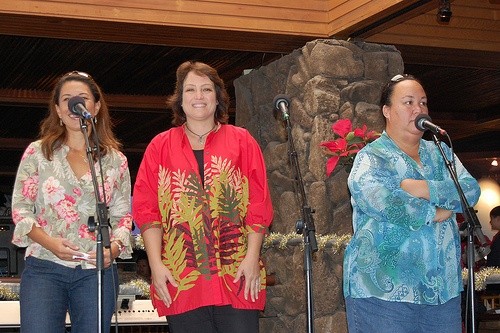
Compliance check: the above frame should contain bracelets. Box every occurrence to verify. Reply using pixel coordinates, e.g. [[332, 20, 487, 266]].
[[115, 242, 122, 251]]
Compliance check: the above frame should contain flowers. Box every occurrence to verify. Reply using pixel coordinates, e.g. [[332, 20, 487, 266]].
[[318, 117, 383, 178]]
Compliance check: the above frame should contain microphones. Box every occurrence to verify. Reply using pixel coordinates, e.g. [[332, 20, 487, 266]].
[[67, 96, 93, 120], [273, 94, 291, 121], [414, 114, 447, 136]]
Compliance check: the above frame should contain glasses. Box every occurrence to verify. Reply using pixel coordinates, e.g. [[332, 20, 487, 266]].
[[384, 74, 409, 92], [63, 70, 93, 81]]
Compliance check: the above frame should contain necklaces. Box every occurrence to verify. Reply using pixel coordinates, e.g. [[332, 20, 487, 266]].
[[185, 123, 215, 143]]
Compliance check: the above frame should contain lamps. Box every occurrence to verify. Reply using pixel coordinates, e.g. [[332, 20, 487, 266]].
[[437, 1, 452, 25]]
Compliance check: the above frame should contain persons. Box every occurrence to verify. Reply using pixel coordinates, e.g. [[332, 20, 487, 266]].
[[342, 75, 480, 333], [135, 254, 151, 282], [10, 71, 134, 333], [486, 206, 500, 296], [132, 61, 273, 333]]
